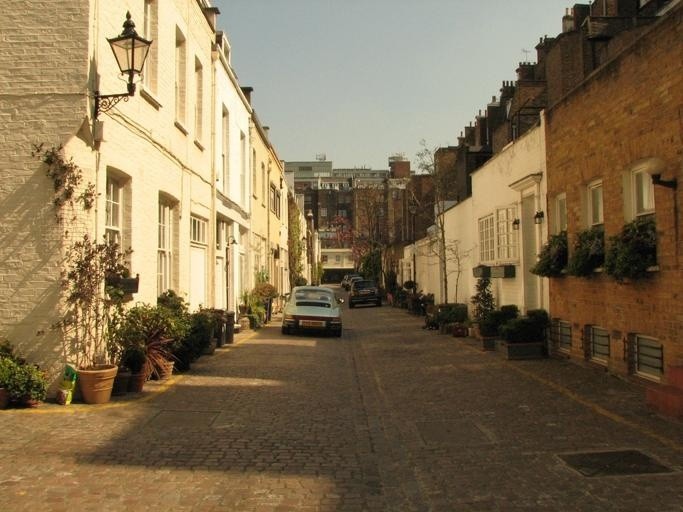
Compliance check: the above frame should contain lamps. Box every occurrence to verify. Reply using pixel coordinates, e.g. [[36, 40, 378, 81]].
[[89, 5, 152, 122], [533, 211, 544, 226], [511, 217, 520, 230], [645, 156, 677, 192]]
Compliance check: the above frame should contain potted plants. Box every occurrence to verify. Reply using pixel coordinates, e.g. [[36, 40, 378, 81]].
[[49, 224, 134, 408], [0, 353, 48, 413], [469, 264, 551, 360], [107, 288, 218, 397]]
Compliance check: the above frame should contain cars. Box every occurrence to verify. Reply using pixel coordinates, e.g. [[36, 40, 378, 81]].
[[341, 274, 363, 291], [280, 286, 345, 336], [348, 280, 382, 308]]
